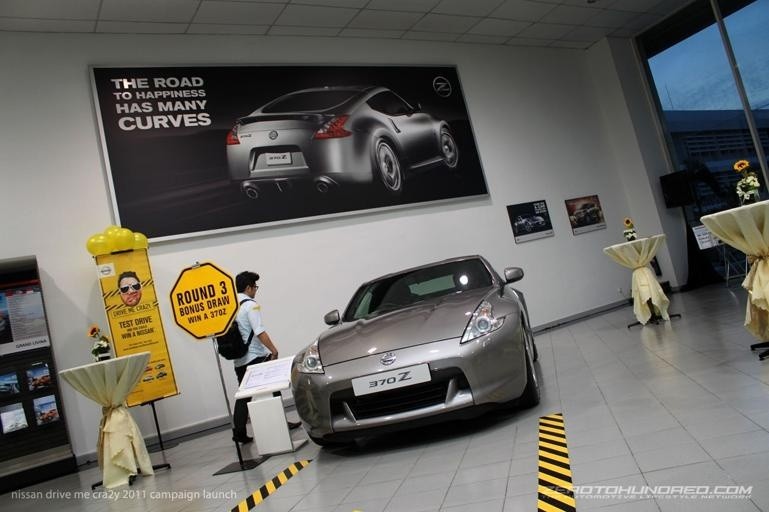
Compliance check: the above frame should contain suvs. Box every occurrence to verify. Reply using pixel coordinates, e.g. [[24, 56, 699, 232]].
[[574, 202, 600, 222]]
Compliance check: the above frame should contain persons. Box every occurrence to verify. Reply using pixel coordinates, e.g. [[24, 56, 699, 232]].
[[217, 270, 302, 444], [117, 270, 141, 305]]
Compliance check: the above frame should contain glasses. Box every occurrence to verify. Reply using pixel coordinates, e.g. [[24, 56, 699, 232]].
[[120, 283, 141, 293]]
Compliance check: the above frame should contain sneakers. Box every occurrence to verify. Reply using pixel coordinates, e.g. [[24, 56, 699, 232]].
[[288, 421, 302, 428], [232, 436, 253, 443]]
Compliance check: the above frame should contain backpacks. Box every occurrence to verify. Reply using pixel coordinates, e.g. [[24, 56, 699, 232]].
[[217, 298, 257, 359]]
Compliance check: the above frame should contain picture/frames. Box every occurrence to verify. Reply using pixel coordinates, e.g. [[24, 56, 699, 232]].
[[83, 58, 495, 245]]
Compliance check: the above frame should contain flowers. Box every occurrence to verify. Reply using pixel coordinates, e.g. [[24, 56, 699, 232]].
[[622, 216, 639, 238], [86, 323, 113, 360], [732, 159, 764, 198]]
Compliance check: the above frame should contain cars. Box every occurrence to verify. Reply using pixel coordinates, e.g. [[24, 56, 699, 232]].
[[0, 383, 12, 392], [290, 254, 541, 450], [225, 85, 460, 203], [31, 375, 48, 387], [40, 409, 57, 423], [515, 214, 546, 233]]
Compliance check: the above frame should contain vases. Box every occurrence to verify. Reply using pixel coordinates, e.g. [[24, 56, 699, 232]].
[[95, 353, 112, 362], [626, 233, 637, 241], [739, 191, 759, 205]]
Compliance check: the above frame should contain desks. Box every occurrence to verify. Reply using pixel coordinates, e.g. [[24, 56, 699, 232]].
[[698, 199, 769, 361], [599, 231, 684, 330], [57, 350, 176, 492]]
